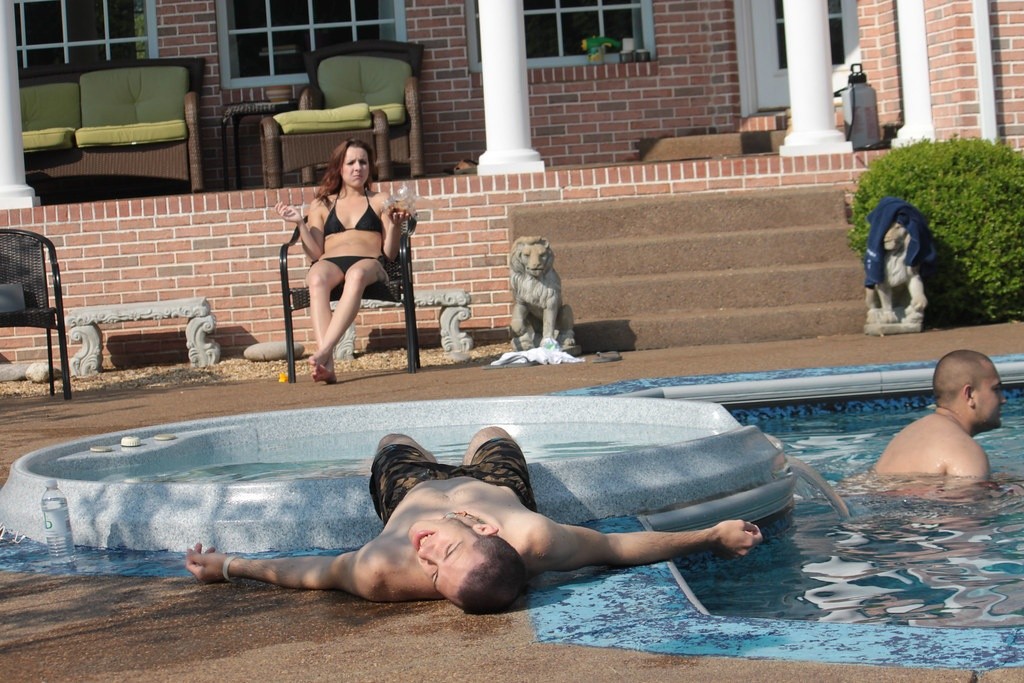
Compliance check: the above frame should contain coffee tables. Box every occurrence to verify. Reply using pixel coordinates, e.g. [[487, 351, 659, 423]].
[[222, 99, 297, 188]]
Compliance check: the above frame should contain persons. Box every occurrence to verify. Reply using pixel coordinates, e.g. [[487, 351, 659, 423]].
[[184, 426, 763, 615], [276, 137, 414, 386], [875, 350, 1007, 485]]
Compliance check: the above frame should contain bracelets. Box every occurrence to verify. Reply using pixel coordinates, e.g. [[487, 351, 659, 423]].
[[223, 555, 243, 581]]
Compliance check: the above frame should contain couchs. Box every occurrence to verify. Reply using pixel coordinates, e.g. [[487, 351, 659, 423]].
[[19, 57, 205, 194], [296, 39, 423, 187]]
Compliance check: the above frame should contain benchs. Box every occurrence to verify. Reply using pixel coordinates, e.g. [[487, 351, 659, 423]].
[[303, 289, 475, 365], [66, 295, 222, 378]]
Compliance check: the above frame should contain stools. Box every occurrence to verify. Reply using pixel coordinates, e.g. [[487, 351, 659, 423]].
[[259, 103, 390, 188]]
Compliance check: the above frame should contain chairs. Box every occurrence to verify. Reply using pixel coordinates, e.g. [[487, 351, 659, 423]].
[[0, 229, 73, 401], [278, 208, 421, 385]]
